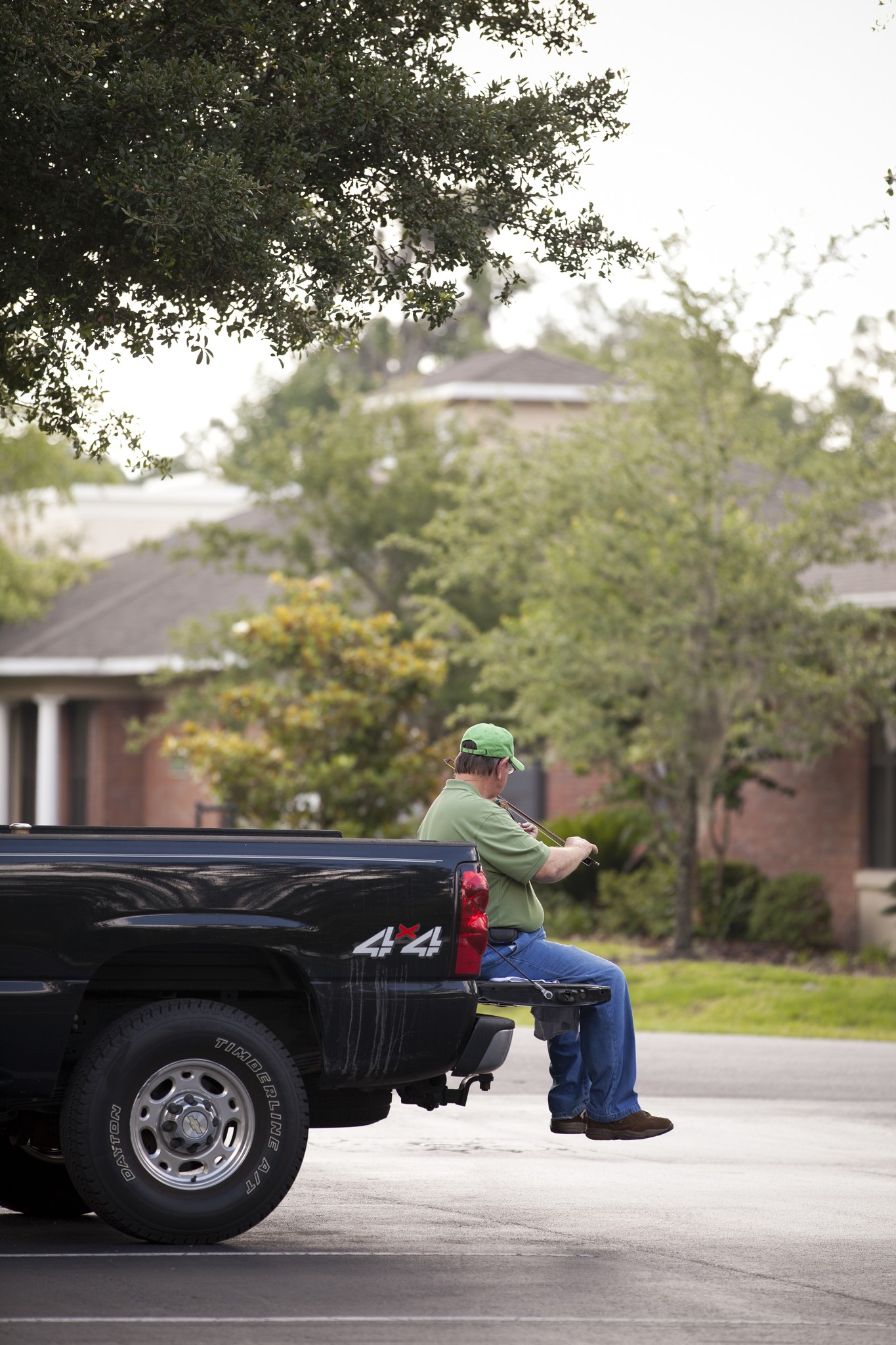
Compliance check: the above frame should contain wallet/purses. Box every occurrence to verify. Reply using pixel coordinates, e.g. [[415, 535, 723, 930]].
[[487, 927, 517, 945]]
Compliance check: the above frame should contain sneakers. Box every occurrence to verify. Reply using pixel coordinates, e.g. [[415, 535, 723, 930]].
[[550, 1109, 586, 1134], [585, 1110, 673, 1140]]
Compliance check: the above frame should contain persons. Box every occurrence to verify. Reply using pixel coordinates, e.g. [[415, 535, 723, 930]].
[[412, 722, 673, 1141]]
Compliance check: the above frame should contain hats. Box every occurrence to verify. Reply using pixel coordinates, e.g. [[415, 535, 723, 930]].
[[460, 724, 525, 771]]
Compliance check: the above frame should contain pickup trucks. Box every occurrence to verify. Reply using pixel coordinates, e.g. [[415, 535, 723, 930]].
[[2, 824, 611, 1246]]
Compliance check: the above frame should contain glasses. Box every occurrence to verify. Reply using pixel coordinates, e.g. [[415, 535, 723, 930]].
[[498, 758, 516, 775]]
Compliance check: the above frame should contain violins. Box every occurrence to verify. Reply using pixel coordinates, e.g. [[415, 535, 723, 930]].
[[494, 797, 516, 821]]
